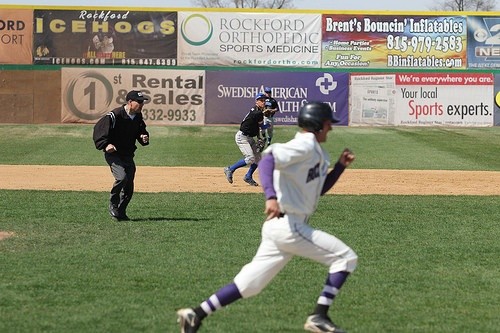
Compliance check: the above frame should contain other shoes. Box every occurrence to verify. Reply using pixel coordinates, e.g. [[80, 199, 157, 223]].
[[223, 166, 233, 183], [112, 214, 129, 221], [243, 176, 258, 186], [108, 204, 119, 217]]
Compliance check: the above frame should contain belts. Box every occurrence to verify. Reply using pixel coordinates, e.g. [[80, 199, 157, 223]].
[[277, 212, 285, 217]]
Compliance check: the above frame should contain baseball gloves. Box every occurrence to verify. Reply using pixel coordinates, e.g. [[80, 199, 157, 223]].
[[264, 109, 274, 116], [256, 139, 265, 152]]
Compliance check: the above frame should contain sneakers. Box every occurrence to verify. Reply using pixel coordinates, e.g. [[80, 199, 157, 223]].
[[177, 307, 203, 333], [304, 313, 345, 333]]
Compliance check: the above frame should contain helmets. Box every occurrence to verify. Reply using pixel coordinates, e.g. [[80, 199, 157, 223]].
[[298, 100, 341, 131]]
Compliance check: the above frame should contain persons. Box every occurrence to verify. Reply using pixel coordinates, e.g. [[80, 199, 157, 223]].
[[223, 93, 272, 187], [93, 90, 150, 219], [175, 102, 358, 333], [261, 87, 280, 146]]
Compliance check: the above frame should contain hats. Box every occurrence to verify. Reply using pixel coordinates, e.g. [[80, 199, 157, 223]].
[[126, 90, 148, 100], [265, 87, 271, 92], [255, 93, 266, 100]]
